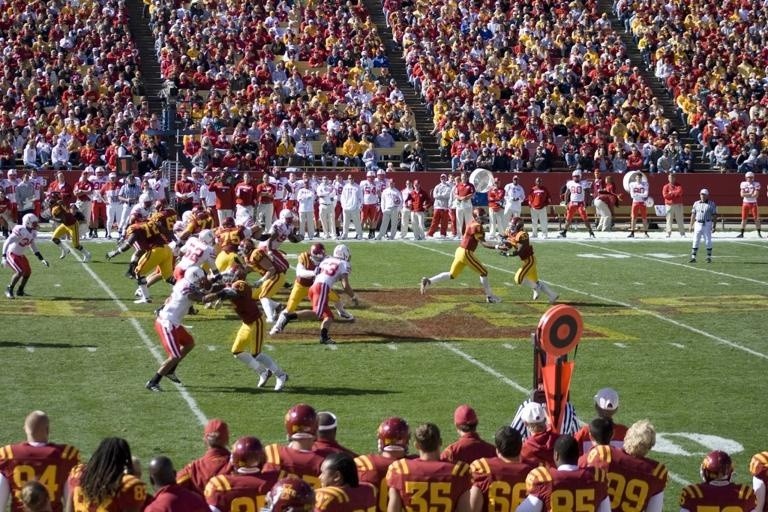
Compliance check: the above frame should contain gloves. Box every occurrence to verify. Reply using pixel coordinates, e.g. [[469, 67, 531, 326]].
[[1, 259, 8, 268], [41, 260, 49, 267]]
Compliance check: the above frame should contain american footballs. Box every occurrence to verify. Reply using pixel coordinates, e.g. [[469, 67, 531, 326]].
[[498, 242, 512, 251]]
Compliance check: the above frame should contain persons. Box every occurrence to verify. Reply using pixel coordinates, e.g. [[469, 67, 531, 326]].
[[0, 0, 768, 394], [146, 230, 361, 393], [1, 305, 767, 511]]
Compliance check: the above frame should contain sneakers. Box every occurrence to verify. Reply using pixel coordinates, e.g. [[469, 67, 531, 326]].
[[5, 283, 15, 299], [547, 292, 560, 302], [258, 368, 272, 387], [687, 258, 696, 264], [81, 233, 124, 246], [145, 380, 163, 392], [705, 258, 712, 264], [758, 234, 762, 237], [339, 312, 353, 320], [736, 234, 743, 238], [105, 250, 116, 260], [420, 277, 429, 295], [60, 248, 70, 259], [126, 272, 152, 304], [275, 373, 288, 391], [627, 234, 688, 239], [487, 295, 503, 303], [529, 231, 596, 239], [309, 233, 461, 240], [83, 252, 91, 263], [320, 335, 336, 344], [533, 281, 542, 300], [266, 304, 288, 336], [16, 290, 32, 296], [165, 369, 181, 383]]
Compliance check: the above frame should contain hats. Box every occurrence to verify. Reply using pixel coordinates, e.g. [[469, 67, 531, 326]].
[[454, 405, 475, 425], [205, 419, 228, 444], [109, 172, 151, 180], [494, 177, 500, 182], [519, 403, 546, 423], [699, 189, 709, 196], [593, 388, 621, 411]]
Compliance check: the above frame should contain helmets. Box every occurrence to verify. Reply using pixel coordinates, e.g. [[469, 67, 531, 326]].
[[508, 216, 523, 234], [572, 170, 582, 182], [285, 404, 318, 440], [231, 435, 268, 473], [745, 171, 755, 180], [262, 477, 315, 511], [698, 450, 732, 482], [333, 244, 351, 261], [366, 170, 376, 181], [223, 266, 247, 286], [22, 213, 39, 231], [472, 207, 490, 225], [272, 166, 282, 179], [0, 166, 105, 179], [184, 266, 206, 289], [376, 417, 412, 451], [191, 167, 201, 179], [377, 168, 386, 177], [279, 209, 295, 224], [130, 192, 169, 222], [310, 242, 325, 259], [182, 205, 255, 257]]
